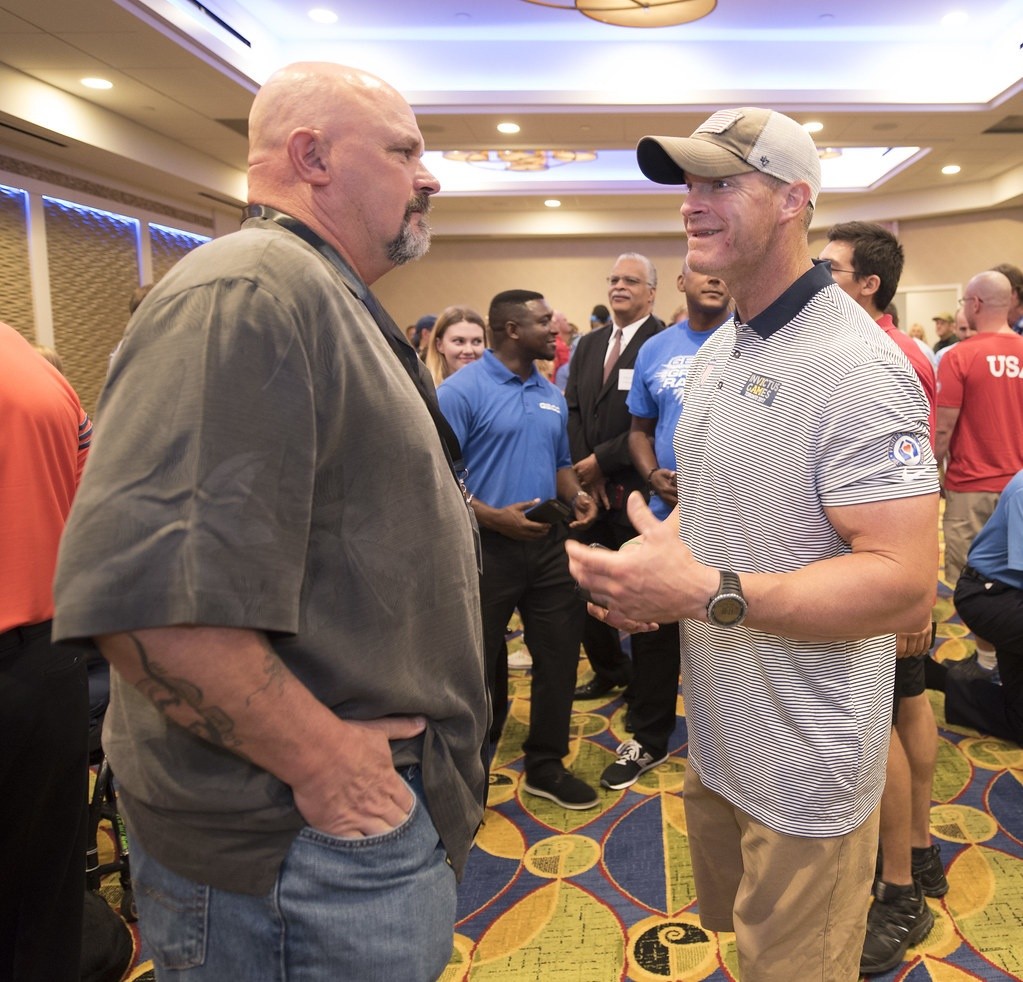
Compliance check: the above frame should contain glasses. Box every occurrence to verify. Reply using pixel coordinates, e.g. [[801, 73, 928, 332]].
[[959, 296, 984, 306], [607, 275, 655, 290]]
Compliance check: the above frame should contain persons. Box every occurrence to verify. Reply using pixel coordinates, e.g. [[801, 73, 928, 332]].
[[0, 318, 106, 982], [820, 221, 1023, 976], [50, 59, 491, 981], [567, 107, 938, 982], [404, 249, 736, 812]]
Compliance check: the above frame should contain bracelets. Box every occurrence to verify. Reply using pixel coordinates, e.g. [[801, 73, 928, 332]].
[[648, 467, 659, 495]]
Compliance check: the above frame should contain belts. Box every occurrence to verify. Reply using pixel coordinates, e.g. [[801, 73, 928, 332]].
[[960, 566, 991, 585], [0, 617, 53, 652]]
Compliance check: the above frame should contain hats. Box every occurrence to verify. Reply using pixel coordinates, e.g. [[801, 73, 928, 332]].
[[933, 313, 954, 324], [636, 104, 819, 203]]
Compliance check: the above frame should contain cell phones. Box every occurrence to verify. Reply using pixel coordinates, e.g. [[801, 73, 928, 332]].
[[524, 499, 571, 525]]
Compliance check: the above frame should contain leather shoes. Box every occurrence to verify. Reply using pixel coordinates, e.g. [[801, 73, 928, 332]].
[[574, 667, 632, 700]]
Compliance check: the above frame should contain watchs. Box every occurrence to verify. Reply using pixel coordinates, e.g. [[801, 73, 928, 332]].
[[704, 569, 749, 630]]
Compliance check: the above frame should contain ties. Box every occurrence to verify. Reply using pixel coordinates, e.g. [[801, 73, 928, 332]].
[[601, 329, 623, 384]]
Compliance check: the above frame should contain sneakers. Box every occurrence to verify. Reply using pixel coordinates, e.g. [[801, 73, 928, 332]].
[[858, 870, 936, 973], [601, 738, 671, 790], [525, 762, 600, 810], [876, 846, 950, 897]]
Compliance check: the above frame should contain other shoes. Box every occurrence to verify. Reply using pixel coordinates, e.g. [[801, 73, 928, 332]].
[[505, 650, 534, 669], [944, 651, 1001, 687]]
